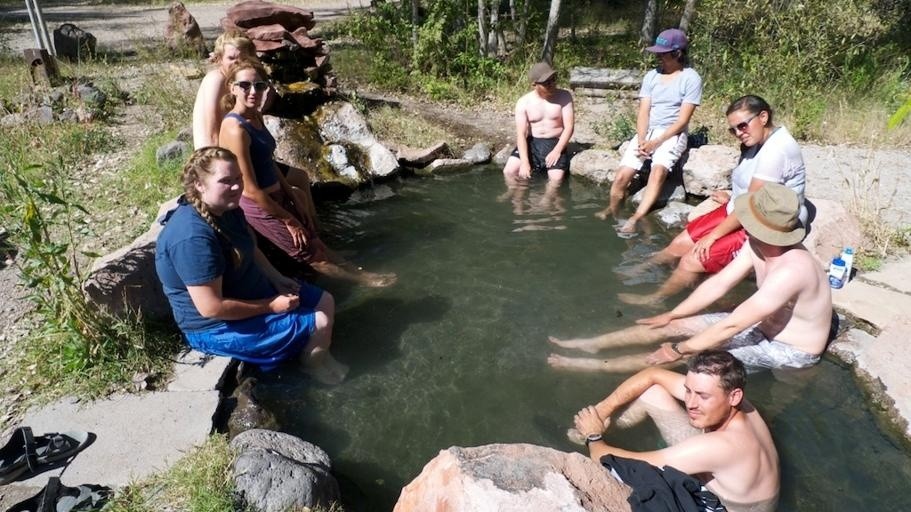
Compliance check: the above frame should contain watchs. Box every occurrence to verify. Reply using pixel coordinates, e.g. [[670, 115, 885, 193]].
[[583, 432, 605, 448]]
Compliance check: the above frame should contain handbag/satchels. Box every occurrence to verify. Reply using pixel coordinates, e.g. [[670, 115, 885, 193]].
[[54, 24, 96, 62]]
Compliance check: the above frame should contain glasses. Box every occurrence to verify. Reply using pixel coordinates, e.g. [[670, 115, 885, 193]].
[[728, 115, 757, 134], [234, 81, 266, 90]]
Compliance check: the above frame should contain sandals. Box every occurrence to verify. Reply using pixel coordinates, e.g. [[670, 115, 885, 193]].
[[0, 427, 89, 484], [6, 477, 113, 512]]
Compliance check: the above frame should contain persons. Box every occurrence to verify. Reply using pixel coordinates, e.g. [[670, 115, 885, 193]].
[[190, 30, 318, 228], [572, 349, 783, 511], [153, 145, 350, 389], [593, 27, 705, 234], [545, 180, 834, 374], [611, 95, 811, 312], [217, 57, 399, 290], [502, 62, 577, 187]]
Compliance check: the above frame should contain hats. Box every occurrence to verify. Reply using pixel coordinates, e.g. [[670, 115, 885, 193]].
[[734, 184, 807, 247], [527, 63, 559, 84], [645, 28, 689, 54]]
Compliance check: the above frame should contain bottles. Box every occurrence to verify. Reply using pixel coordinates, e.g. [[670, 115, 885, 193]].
[[829, 258, 847, 284], [841, 247, 853, 285]]
